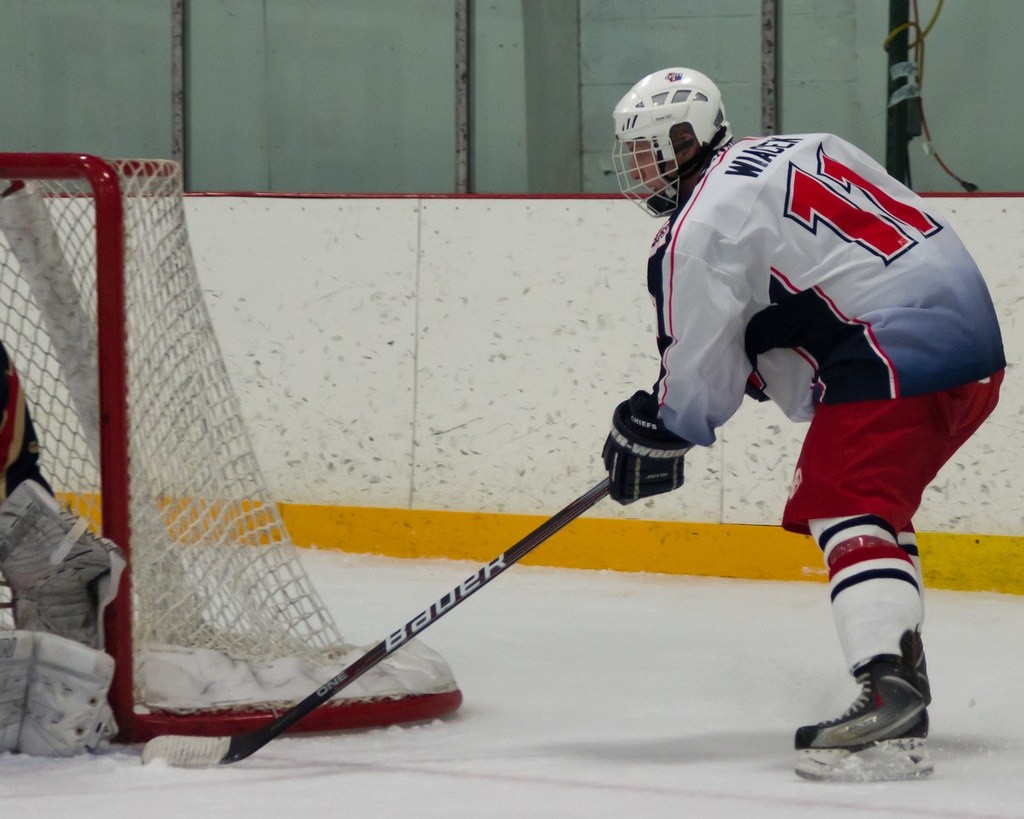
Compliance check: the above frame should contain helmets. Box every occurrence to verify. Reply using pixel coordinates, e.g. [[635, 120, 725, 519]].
[[612, 66, 734, 161]]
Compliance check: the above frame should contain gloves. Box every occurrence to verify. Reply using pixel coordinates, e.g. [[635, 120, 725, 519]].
[[601, 389, 694, 506]]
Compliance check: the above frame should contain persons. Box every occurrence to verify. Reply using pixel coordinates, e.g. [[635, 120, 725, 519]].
[[615, 61, 1006, 750]]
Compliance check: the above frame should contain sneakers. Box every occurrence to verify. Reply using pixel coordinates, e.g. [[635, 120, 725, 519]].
[[793, 623, 934, 781]]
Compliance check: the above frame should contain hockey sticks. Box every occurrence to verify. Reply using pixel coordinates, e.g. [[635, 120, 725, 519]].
[[142, 478, 613, 768]]
